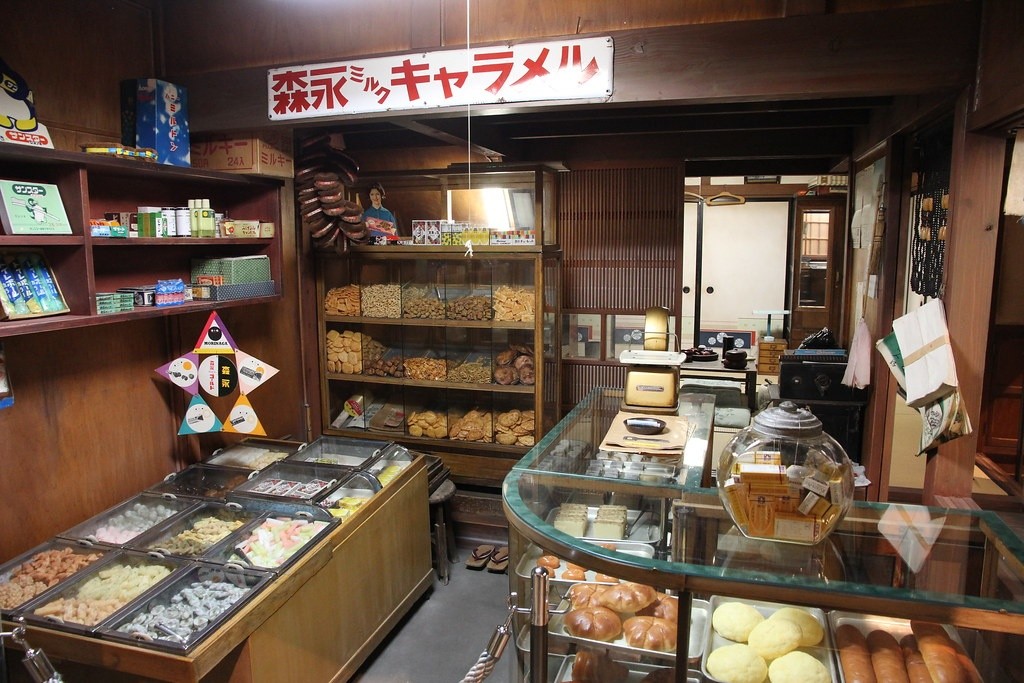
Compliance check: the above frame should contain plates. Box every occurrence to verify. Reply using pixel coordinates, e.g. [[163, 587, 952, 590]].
[[623, 418, 666, 434]]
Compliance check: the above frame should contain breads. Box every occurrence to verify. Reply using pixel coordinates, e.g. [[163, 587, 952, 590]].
[[834, 617, 984, 683], [532, 553, 679, 652], [551, 501, 627, 539]]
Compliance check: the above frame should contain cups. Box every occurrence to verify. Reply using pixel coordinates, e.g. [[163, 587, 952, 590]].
[[682, 349, 692, 362]]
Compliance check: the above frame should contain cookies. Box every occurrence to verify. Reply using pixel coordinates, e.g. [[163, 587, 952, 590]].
[[406, 407, 536, 447], [326, 330, 385, 375], [496, 340, 536, 386]]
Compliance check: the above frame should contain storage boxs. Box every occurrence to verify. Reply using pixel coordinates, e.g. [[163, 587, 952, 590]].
[[0, 248, 71, 322], [412, 219, 535, 246], [0, 179, 73, 236], [116, 285, 193, 307], [190, 127, 294, 179], [191, 257, 270, 284], [187, 281, 275, 301], [136, 79, 191, 167]]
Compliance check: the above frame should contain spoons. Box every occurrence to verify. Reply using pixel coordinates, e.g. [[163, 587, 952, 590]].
[[624, 436, 669, 443]]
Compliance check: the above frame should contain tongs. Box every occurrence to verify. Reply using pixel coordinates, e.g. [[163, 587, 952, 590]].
[[625, 503, 654, 540]]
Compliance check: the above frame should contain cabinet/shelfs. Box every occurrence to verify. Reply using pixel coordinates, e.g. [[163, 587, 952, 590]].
[[315, 250, 561, 481], [0, 141, 286, 338]]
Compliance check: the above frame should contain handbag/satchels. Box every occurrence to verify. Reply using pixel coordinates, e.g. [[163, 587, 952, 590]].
[[797, 327, 840, 349]]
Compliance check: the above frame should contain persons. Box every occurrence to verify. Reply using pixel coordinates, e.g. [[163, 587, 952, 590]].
[[361, 182, 397, 240]]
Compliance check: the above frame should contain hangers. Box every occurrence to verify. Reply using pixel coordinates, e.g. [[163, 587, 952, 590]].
[[706, 184, 745, 205], [684, 191, 703, 202]]
